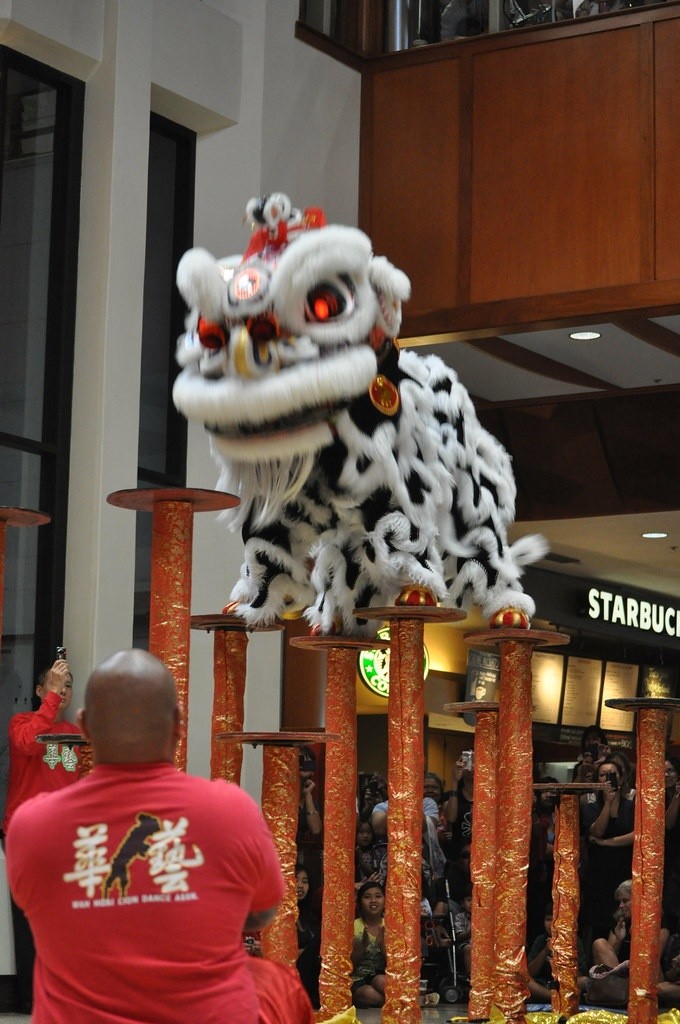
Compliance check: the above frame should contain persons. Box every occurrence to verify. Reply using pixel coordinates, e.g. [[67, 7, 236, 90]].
[[5, 648, 315, 1024], [0, 659, 89, 1016], [295, 724, 680, 1011], [413, 0, 668, 55]]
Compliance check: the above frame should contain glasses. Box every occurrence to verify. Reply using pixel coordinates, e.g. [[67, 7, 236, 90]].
[[665, 768, 677, 775]]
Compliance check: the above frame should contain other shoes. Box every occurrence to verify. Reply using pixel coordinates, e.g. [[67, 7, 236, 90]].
[[425, 992, 440, 1007]]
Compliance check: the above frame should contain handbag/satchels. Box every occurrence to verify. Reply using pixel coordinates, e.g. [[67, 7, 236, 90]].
[[585, 960, 630, 1006]]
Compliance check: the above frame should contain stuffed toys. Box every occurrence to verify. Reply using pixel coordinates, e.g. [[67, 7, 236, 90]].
[[171, 190, 548, 636]]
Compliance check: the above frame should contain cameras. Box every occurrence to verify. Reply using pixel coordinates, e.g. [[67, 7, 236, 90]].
[[57, 646, 66, 661]]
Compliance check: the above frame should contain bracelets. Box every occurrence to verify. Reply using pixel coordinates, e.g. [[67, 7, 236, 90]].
[[448, 791, 458, 796]]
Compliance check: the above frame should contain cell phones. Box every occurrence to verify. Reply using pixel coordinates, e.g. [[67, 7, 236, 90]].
[[587, 742, 598, 761], [605, 771, 618, 792], [370, 781, 379, 794], [461, 751, 471, 772]]
